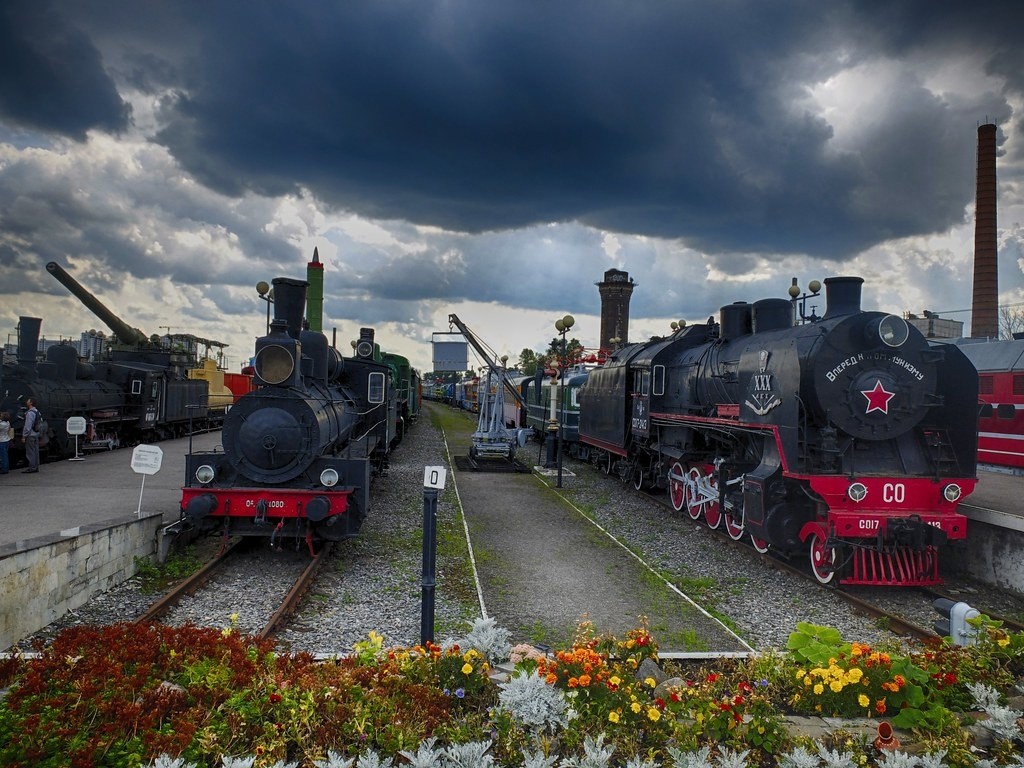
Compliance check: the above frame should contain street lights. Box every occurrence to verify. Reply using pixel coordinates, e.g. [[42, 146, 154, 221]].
[[555, 315, 575, 488], [609, 337, 620, 351], [789, 280, 821, 324], [500, 355, 508, 368]]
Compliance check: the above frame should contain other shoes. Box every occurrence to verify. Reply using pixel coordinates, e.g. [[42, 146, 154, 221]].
[[21, 469, 40, 473], [0, 471, 8, 474]]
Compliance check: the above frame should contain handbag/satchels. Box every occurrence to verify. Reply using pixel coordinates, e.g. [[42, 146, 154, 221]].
[[7, 428, 15, 439]]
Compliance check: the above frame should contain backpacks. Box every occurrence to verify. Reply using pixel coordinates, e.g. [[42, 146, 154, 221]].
[[33, 410, 48, 434]]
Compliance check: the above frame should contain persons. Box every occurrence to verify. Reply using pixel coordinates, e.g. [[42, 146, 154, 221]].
[[0, 412, 10, 474], [21, 398, 39, 473]]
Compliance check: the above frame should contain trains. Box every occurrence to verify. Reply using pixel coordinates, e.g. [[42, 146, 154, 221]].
[[424, 275, 980, 588], [0, 261, 261, 465], [926, 332, 1024, 472], [176, 243, 423, 541]]
